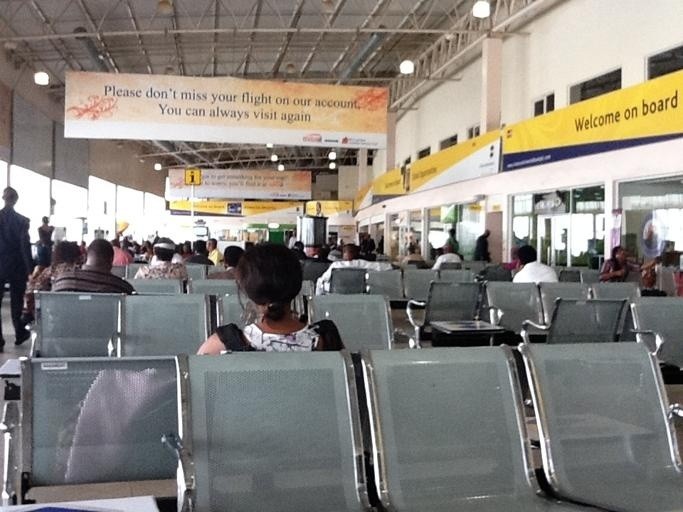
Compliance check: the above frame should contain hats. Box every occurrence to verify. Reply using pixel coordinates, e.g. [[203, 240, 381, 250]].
[[153, 238, 175, 250]]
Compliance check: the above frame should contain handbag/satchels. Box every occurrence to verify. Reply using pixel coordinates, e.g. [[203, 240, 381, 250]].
[[641, 266, 656, 288]]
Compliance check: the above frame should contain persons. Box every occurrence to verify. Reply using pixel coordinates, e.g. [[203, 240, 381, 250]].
[[1, 186, 345, 353], [511, 239, 655, 284], [360, 229, 491, 270], [317, 244, 393, 282]]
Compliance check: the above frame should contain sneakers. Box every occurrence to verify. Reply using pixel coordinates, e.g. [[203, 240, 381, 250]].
[[14, 331, 30, 344], [0, 340, 5, 352]]
